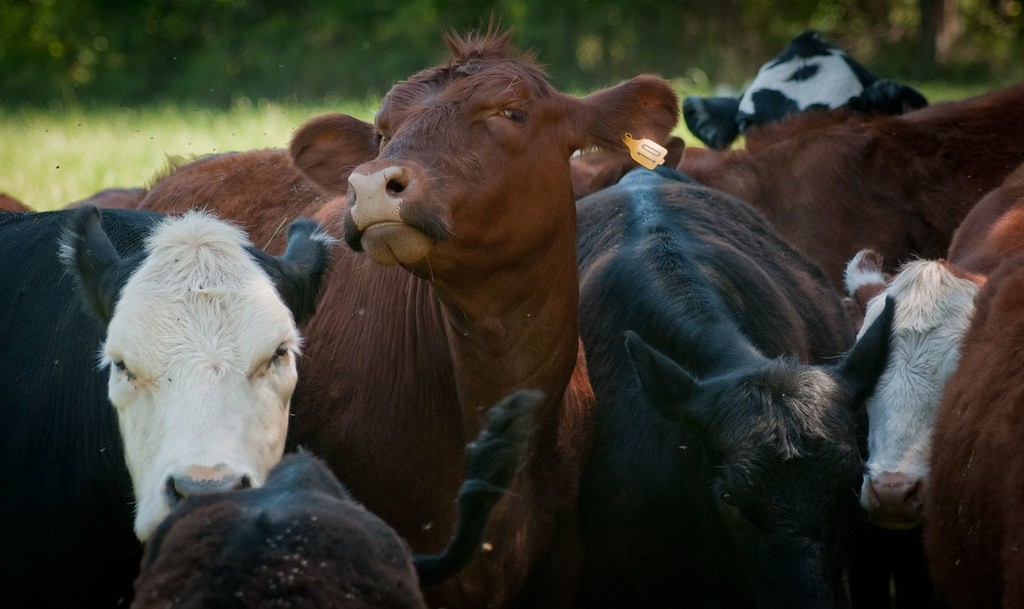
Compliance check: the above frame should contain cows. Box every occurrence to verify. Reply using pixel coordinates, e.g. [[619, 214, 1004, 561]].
[[2, 34, 1024, 609]]
